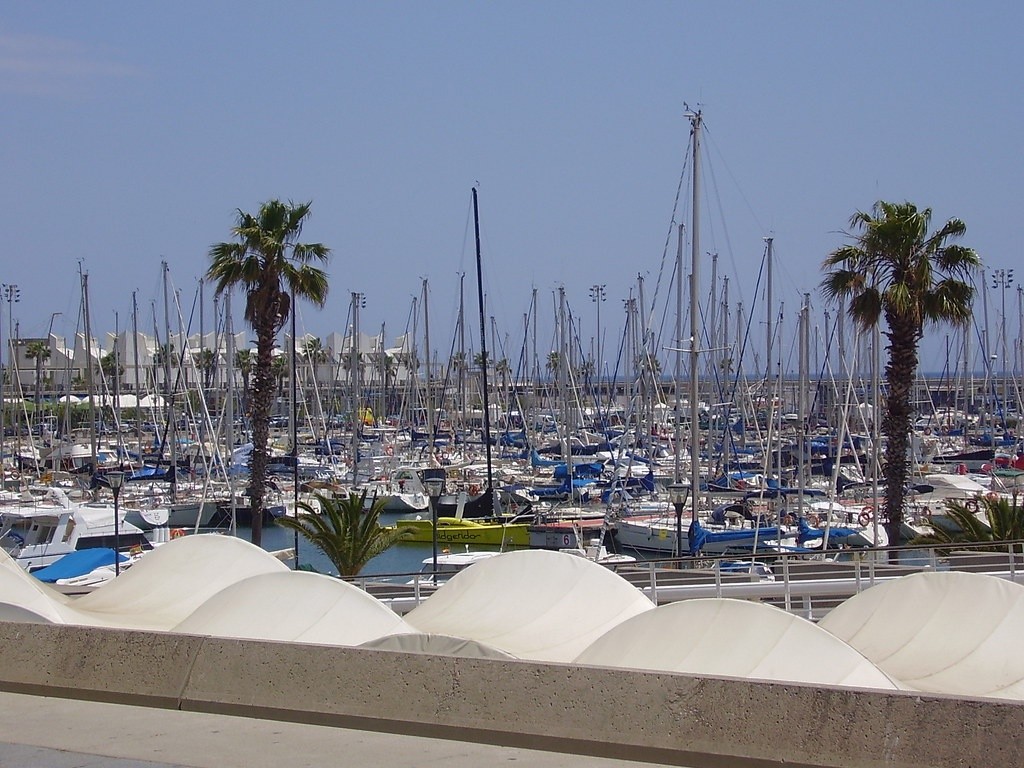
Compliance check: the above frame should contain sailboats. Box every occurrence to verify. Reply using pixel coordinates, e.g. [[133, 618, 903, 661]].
[[0, 98, 1024, 615]]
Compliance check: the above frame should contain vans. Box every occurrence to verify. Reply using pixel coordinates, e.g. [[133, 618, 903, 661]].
[[536, 415, 556, 426]]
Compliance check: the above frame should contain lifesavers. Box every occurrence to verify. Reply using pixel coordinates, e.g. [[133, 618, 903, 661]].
[[170, 529, 185, 540], [924, 427, 932, 436], [10, 470, 20, 480], [385, 446, 394, 457], [861, 505, 874, 522], [807, 516, 819, 527], [784, 515, 792, 525], [858, 512, 869, 526], [469, 485, 478, 496], [965, 501, 979, 514]]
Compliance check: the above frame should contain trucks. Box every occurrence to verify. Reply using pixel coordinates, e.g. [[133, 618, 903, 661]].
[[564, 408, 596, 428]]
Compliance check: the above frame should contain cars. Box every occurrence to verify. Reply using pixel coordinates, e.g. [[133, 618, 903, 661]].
[[4, 423, 34, 435], [76, 421, 91, 430], [174, 418, 198, 430], [234, 416, 249, 428], [144, 421, 159, 432], [32, 423, 54, 435], [270, 417, 283, 427]]
